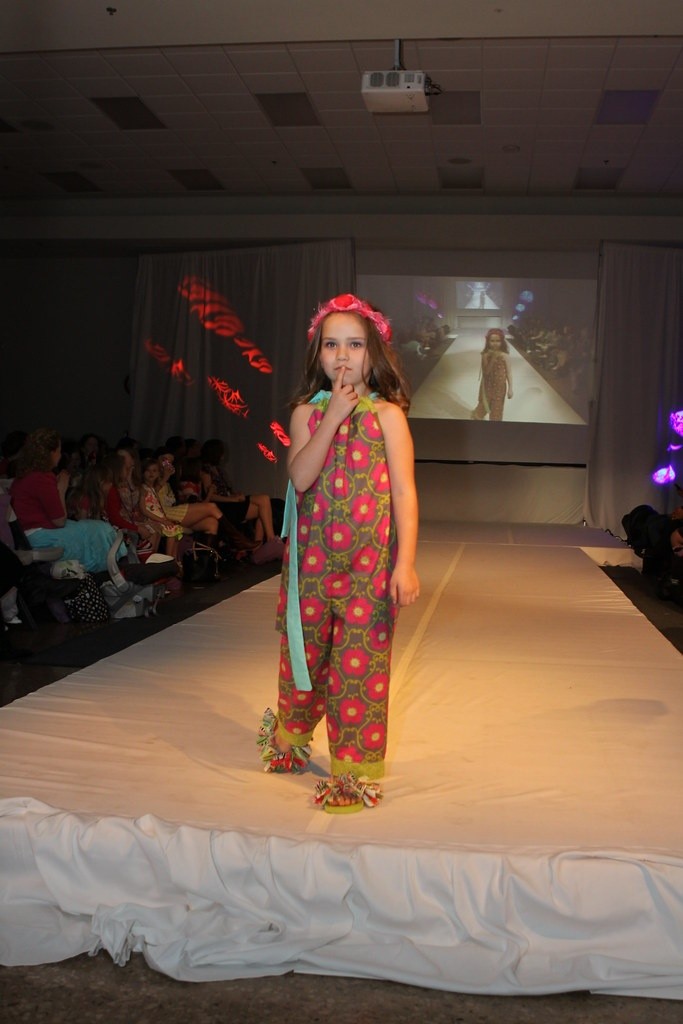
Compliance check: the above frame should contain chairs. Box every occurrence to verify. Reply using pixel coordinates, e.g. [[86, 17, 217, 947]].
[[0, 476, 64, 632]]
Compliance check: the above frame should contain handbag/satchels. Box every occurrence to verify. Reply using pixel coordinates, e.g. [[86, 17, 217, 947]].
[[99, 528, 157, 618], [63, 575, 109, 623]]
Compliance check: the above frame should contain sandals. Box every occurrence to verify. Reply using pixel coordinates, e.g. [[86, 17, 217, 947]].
[[256, 706, 312, 774], [314, 770, 382, 813]]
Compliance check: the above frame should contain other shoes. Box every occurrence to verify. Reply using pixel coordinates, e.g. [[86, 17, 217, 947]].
[[0, 641, 34, 660], [6, 619, 30, 631]]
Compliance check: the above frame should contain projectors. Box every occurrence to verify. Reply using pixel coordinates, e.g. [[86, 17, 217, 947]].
[[361, 70, 429, 113]]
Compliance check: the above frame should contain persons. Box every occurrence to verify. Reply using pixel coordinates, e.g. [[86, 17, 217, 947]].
[[397, 314, 444, 348], [0, 427, 276, 630], [645, 489, 683, 591], [522, 318, 591, 372], [255, 294, 420, 815], [473, 328, 511, 420]]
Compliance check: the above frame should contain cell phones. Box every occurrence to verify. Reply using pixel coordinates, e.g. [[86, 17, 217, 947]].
[[163, 460, 175, 473]]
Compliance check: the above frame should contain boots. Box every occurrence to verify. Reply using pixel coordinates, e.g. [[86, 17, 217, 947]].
[[217, 515, 263, 553], [199, 534, 232, 583]]
